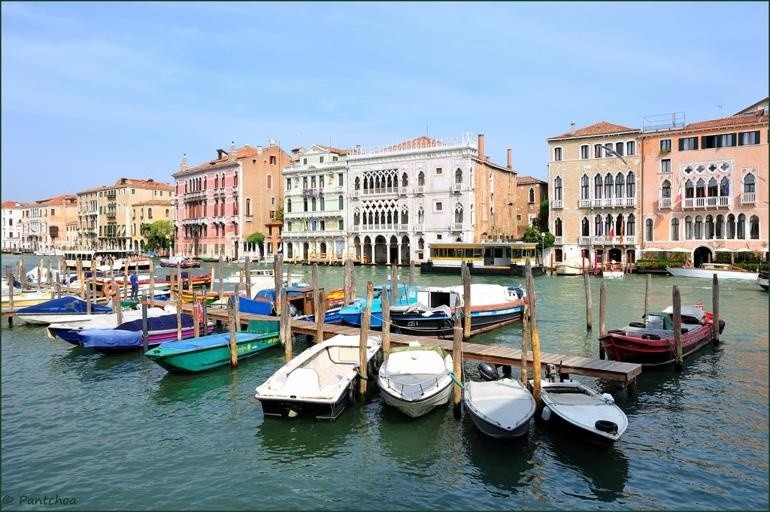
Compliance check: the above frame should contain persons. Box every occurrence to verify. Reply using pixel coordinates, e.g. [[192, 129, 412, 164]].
[[130, 271, 138, 300]]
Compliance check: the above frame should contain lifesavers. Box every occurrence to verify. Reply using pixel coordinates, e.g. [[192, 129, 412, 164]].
[[104, 283, 119, 296]]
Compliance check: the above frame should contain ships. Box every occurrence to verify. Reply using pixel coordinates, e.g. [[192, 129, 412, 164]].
[[428, 243, 539, 275], [64, 248, 151, 271]]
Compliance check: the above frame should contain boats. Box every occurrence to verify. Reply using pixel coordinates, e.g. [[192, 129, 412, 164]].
[[255, 333, 383, 422], [390, 284, 530, 340], [159, 256, 201, 268], [665, 262, 760, 280], [555, 260, 625, 279], [377, 342, 454, 419], [1, 268, 362, 374], [340, 283, 421, 328], [599, 302, 725, 367], [527, 375, 630, 443], [462, 364, 537, 439]]
